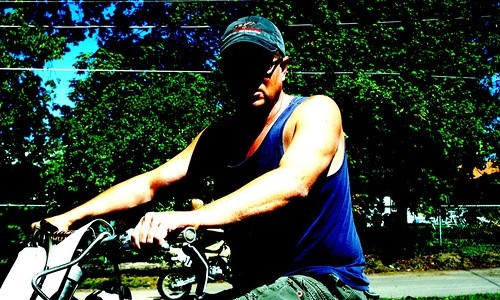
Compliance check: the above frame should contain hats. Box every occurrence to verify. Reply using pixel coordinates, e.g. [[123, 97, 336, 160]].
[[218, 16, 285, 58]]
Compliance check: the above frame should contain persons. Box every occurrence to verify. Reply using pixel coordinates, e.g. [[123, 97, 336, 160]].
[[32, 16, 371, 300]]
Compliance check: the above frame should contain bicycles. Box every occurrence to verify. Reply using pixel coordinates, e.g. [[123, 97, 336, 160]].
[[0, 220, 163, 300]]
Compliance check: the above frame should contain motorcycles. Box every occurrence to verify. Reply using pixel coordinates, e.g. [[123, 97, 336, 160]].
[[158, 226, 232, 300]]
